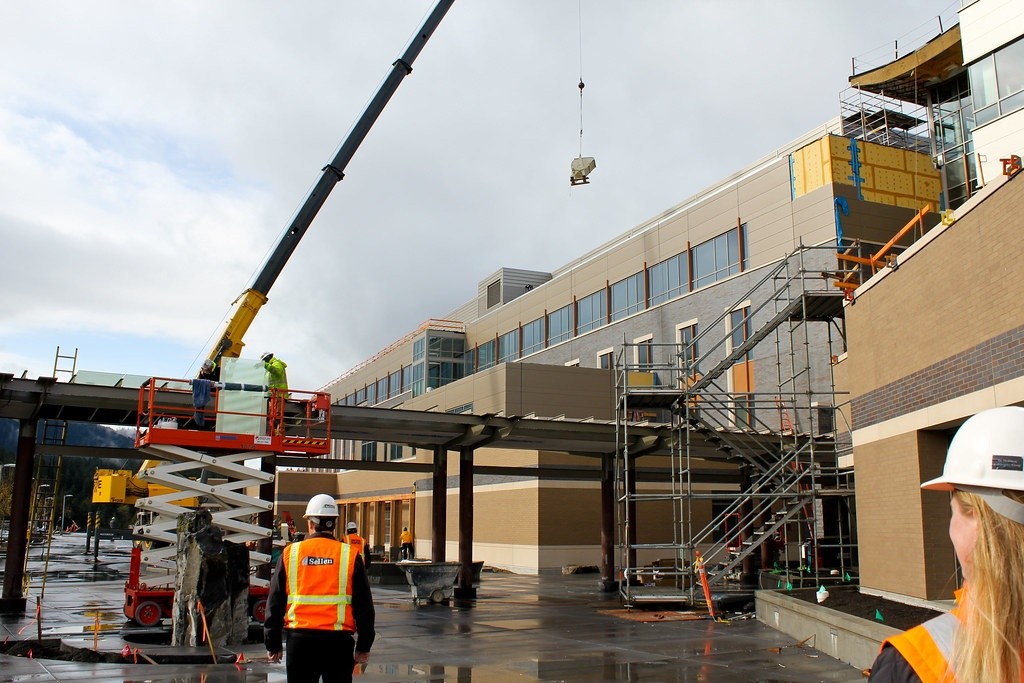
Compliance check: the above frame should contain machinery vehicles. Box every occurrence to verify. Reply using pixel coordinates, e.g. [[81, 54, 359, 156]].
[[91, 1, 598, 625]]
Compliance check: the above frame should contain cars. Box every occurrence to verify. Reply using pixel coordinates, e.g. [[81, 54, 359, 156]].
[[0, 529, 9, 542]]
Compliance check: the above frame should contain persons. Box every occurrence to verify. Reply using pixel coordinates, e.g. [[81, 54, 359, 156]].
[[52, 517, 62, 536], [399, 527, 415, 561], [110, 517, 118, 530], [341, 522, 370, 572], [253, 351, 288, 436], [868, 406, 1024, 683], [264, 494, 376, 683]]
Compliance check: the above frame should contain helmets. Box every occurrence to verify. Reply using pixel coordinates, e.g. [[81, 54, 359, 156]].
[[260, 351, 274, 360], [920, 405, 1024, 490], [347, 522, 357, 529], [303, 494, 339, 519]]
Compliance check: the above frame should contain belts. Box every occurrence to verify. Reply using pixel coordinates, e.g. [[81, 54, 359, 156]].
[[289, 632, 320, 638]]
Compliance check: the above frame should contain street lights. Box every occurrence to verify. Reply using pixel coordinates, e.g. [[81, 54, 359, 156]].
[[61, 495, 73, 534], [36, 484, 50, 493], [43, 497, 52, 529]]
